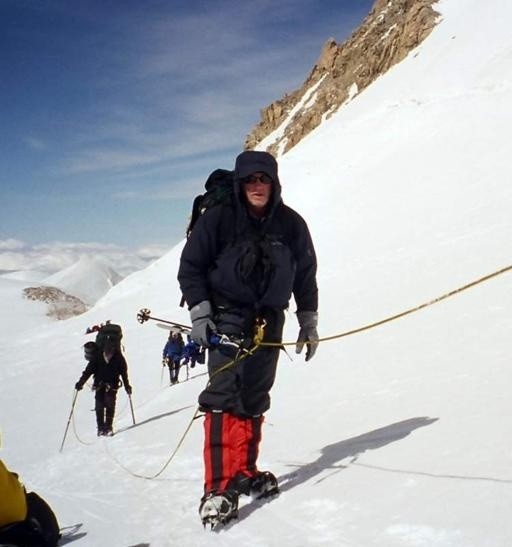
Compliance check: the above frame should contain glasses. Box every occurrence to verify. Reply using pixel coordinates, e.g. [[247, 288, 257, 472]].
[[246, 174, 271, 184]]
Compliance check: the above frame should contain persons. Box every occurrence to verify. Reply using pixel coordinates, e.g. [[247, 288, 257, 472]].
[[162, 331, 189, 384], [74, 340, 131, 436], [177, 150, 319, 520], [0, 459, 62, 547], [183, 335, 200, 368]]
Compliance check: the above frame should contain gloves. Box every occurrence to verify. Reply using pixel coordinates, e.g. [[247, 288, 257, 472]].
[[295, 311, 319, 361], [190, 301, 217, 347], [75, 380, 84, 391], [125, 383, 133, 395]]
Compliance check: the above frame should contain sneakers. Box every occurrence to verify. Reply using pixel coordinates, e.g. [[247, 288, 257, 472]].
[[250, 472, 278, 502], [97, 430, 114, 437], [199, 493, 238, 529]]
